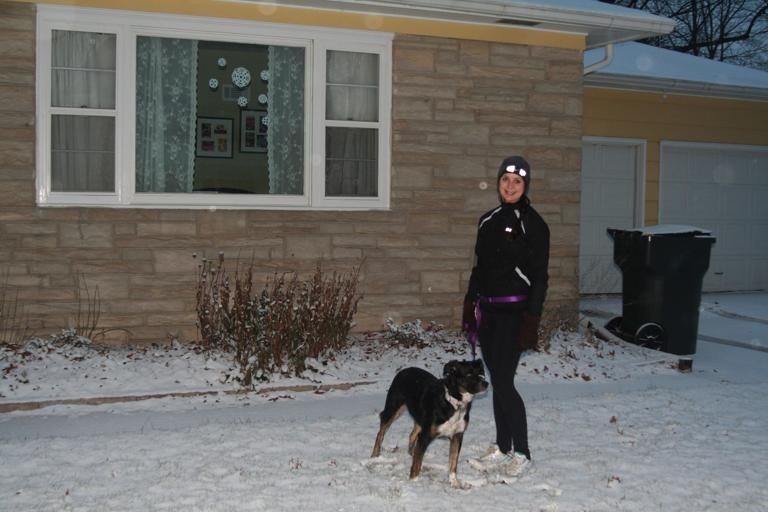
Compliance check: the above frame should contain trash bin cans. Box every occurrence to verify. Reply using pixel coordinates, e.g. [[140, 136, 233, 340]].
[[606, 225, 717, 373]]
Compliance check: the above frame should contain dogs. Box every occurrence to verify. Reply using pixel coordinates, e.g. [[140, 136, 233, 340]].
[[372, 357, 490, 491]]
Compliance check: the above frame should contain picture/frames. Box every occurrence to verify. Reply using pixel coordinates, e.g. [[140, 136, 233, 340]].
[[238, 108, 268, 154], [196, 116, 233, 159]]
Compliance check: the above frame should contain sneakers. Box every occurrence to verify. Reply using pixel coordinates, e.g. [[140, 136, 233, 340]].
[[480, 444, 512, 460], [511, 453, 529, 475]]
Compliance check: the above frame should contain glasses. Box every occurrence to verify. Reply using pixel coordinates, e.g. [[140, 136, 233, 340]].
[[506, 164, 527, 177]]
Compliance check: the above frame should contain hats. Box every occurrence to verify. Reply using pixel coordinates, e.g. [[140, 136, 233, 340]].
[[497, 156, 530, 195]]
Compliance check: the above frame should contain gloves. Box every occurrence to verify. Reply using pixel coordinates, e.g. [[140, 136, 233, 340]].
[[519, 313, 539, 350], [461, 300, 477, 343]]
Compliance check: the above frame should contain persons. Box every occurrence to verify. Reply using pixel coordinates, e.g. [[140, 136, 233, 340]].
[[461, 155, 550, 472]]
